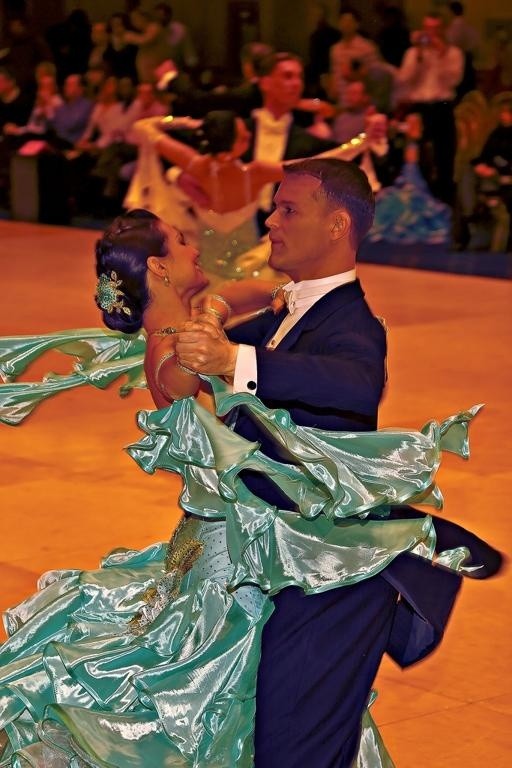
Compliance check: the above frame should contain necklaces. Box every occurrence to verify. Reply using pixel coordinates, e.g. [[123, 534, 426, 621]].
[[152, 327, 176, 334]]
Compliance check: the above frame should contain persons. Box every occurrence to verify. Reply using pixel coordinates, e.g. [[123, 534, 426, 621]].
[[133, 52, 388, 291], [2, 208, 489, 768], [1, 1, 512, 254], [175, 162, 507, 767]]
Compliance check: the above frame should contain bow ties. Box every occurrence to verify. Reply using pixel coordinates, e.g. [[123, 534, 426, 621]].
[[281, 281, 349, 320]]
